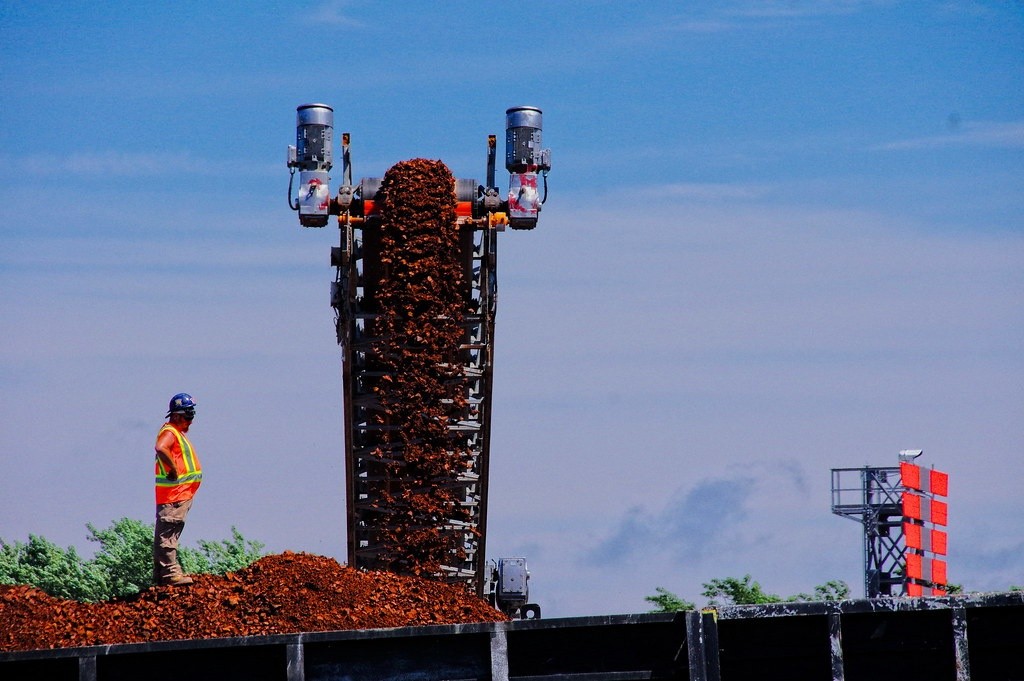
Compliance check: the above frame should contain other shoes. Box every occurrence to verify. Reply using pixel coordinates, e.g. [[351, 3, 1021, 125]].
[[152, 571, 193, 586]]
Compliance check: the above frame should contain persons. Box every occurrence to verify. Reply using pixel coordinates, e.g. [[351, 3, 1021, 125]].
[[152, 393, 203, 585]]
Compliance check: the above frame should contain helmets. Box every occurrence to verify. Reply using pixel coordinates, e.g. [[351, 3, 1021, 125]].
[[167, 393, 196, 412]]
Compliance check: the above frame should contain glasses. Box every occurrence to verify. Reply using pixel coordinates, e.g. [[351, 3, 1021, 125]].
[[176, 413, 195, 421]]
